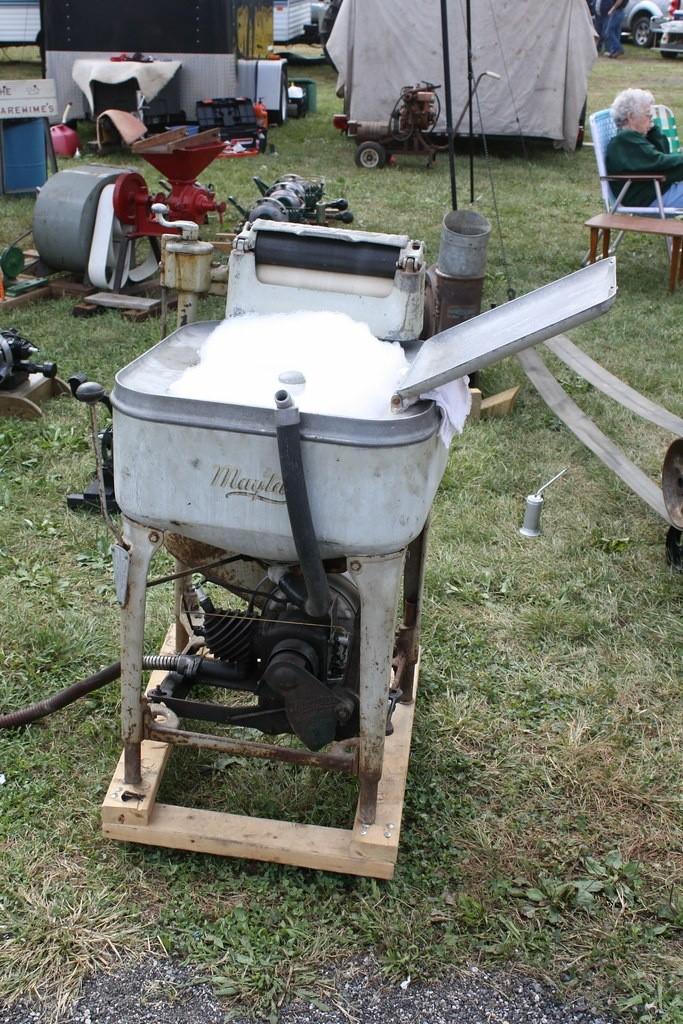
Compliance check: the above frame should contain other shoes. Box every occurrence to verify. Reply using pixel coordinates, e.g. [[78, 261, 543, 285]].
[[604, 50, 612, 56], [612, 50, 624, 58]]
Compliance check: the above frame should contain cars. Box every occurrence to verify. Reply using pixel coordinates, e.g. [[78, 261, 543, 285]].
[[589, 1, 683, 59]]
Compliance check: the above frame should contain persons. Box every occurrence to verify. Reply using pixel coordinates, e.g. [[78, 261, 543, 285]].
[[604, 88, 683, 221], [592, 0, 629, 59]]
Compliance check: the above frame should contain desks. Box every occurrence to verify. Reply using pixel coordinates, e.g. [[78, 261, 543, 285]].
[[587, 213, 683, 292]]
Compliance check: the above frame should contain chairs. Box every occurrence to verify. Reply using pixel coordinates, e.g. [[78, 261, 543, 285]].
[[638, 103, 681, 156], [579, 108, 683, 270]]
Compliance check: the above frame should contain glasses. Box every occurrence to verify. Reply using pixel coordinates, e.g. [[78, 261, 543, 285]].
[[643, 113, 653, 119]]
